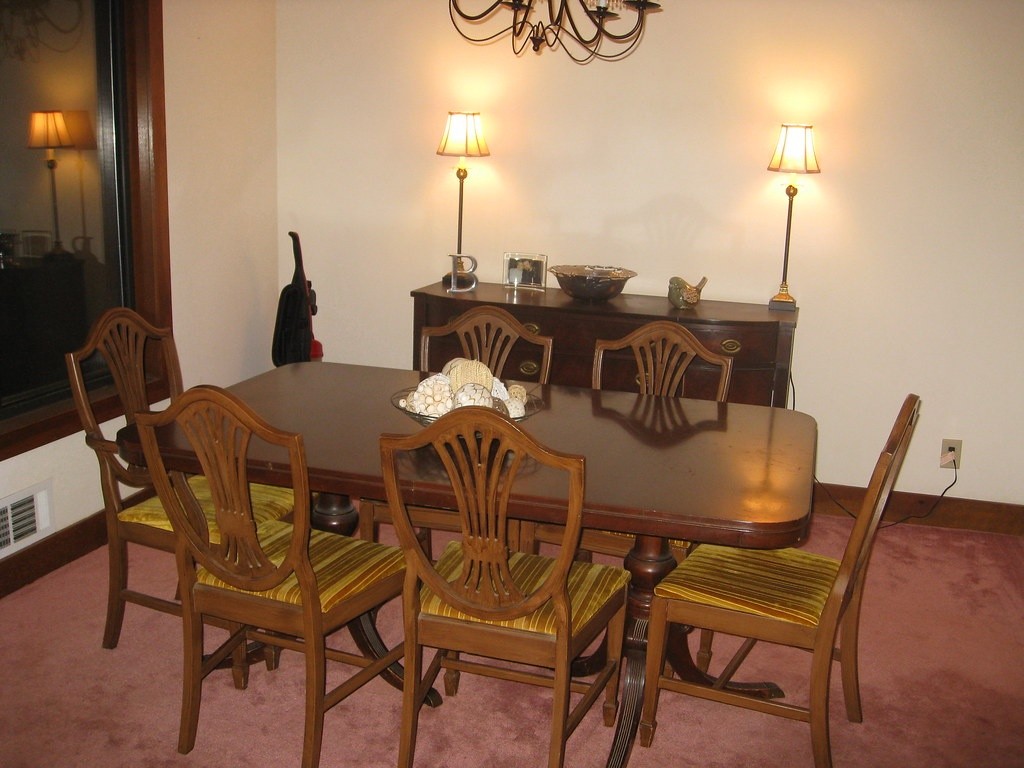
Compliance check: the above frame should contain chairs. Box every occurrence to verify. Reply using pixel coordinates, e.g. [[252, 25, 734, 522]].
[[639, 393, 923, 768], [358, 304, 555, 566], [518, 321, 734, 630], [63, 307, 319, 689], [376, 405, 631, 768], [134, 384, 429, 767]]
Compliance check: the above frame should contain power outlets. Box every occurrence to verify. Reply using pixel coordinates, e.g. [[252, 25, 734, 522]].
[[939, 437, 963, 469]]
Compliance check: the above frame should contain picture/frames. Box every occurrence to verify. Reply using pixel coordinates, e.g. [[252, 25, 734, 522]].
[[503, 251, 548, 292]]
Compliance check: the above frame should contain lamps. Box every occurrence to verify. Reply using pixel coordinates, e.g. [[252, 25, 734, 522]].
[[766, 121, 821, 310], [448, 0, 661, 62], [435, 110, 491, 285], [26, 110, 74, 259]]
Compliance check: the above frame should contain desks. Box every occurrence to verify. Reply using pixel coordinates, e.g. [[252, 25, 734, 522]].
[[116, 358, 820, 768]]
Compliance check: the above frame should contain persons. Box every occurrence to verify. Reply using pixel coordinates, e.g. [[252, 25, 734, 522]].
[[509, 259, 540, 284]]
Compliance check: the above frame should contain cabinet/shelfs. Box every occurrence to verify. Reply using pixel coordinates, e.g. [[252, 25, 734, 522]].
[[407, 277, 801, 410]]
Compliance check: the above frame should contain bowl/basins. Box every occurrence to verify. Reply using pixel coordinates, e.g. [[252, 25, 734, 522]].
[[547, 264, 638, 304], [391, 385, 546, 440]]
